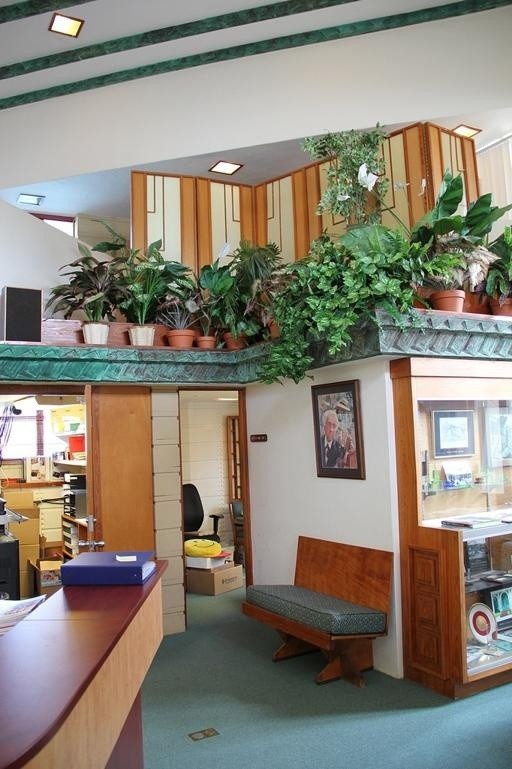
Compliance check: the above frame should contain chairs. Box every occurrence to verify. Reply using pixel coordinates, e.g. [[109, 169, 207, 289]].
[[183, 483, 224, 542]]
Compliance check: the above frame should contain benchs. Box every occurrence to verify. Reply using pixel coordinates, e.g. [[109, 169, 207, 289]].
[[240, 535, 395, 689]]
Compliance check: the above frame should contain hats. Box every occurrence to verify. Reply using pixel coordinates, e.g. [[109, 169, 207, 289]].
[[338, 399, 352, 412]]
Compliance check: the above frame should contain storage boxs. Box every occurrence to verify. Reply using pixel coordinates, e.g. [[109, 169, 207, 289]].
[[185, 566, 244, 595], [185, 552, 231, 569], [186, 561, 234, 573], [27, 557, 64, 594]]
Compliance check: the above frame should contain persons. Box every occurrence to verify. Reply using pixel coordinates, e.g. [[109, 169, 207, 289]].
[[320, 411, 344, 467]]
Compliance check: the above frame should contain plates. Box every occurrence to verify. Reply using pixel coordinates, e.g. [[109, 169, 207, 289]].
[[480, 570, 512, 583], [467, 602, 497, 645]]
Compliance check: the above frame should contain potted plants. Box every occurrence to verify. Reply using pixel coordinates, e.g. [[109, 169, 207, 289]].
[[265, 263, 283, 338], [194, 260, 235, 350], [410, 167, 512, 314], [93, 217, 165, 346], [269, 316, 281, 338], [45, 242, 129, 343], [414, 283, 432, 308], [156, 276, 205, 347], [224, 239, 281, 348]]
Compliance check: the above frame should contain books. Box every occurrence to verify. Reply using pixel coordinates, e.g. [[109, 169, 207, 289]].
[[441, 510, 500, 528]]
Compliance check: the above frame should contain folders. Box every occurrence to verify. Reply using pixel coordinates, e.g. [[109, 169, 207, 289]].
[[58, 546, 156, 586]]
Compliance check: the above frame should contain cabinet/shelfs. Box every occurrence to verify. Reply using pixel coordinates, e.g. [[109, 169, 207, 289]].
[[390, 357, 512, 700], [61, 515, 87, 558]]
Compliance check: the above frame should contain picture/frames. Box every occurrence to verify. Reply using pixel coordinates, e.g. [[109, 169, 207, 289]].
[[311, 379, 364, 479], [431, 409, 476, 460], [478, 408, 512, 467], [483, 584, 512, 632]]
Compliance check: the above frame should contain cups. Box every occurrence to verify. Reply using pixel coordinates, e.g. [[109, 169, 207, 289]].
[[485, 623, 498, 654]]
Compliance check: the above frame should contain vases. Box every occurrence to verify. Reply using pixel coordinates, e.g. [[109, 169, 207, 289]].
[[488, 226, 512, 314]]
